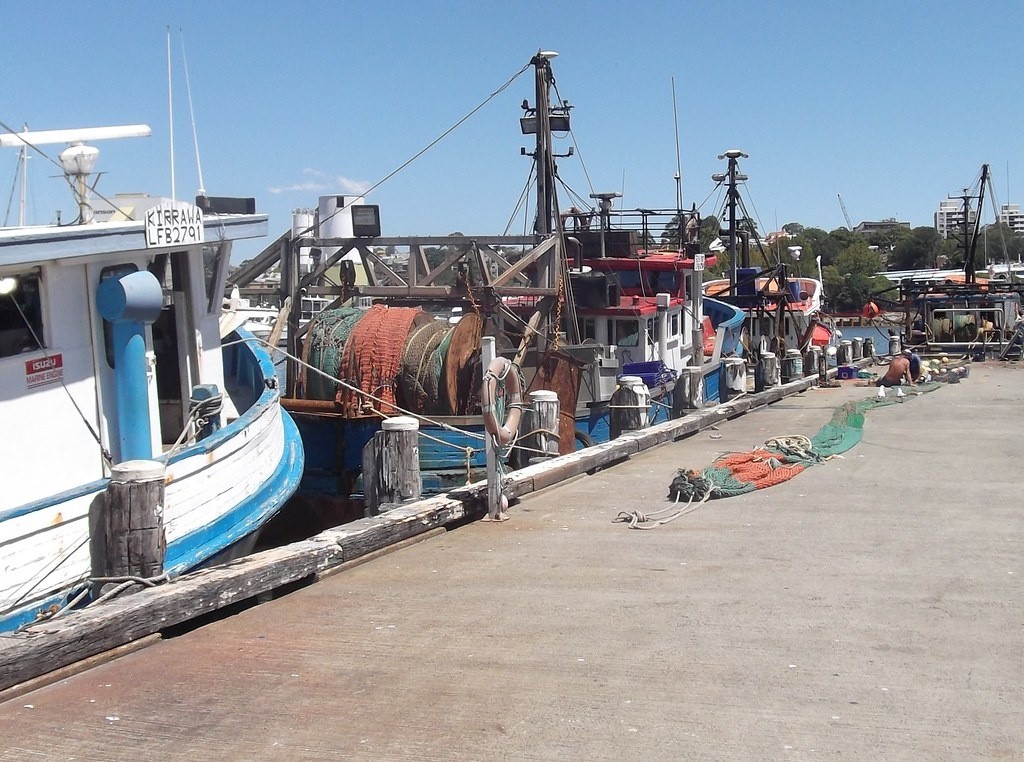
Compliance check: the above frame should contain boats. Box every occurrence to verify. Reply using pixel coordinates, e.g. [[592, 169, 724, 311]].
[[224, 47, 825, 536], [0, 127, 306, 637], [865, 161, 1024, 347]]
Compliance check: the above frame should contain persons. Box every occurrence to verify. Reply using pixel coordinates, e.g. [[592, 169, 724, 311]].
[[868, 349, 931, 387]]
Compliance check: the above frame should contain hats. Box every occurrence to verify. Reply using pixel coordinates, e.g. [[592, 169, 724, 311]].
[[902, 349, 912, 356]]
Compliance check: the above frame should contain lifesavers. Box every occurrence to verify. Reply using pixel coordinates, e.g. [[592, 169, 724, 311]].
[[480, 357, 523, 443], [684, 217, 700, 244], [560, 208, 589, 231]]
